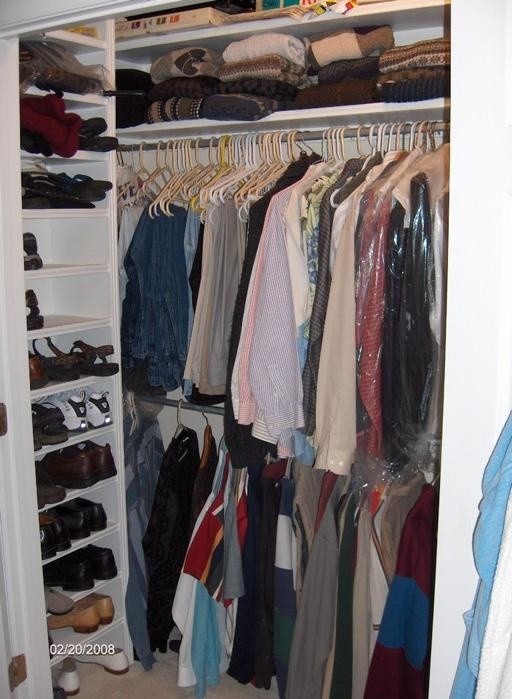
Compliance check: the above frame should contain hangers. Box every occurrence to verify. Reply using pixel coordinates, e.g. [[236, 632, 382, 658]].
[[113, 119, 446, 227], [130, 389, 275, 492]]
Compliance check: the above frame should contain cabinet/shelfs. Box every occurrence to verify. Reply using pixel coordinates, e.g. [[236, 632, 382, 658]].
[[20, 32, 130, 698]]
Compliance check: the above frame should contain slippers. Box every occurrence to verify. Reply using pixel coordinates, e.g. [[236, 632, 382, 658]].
[[76, 117, 118, 153], [22, 175, 111, 208]]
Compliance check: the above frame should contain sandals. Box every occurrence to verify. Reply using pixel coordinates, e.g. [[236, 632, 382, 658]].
[[26, 290, 44, 329], [24, 232, 43, 270]]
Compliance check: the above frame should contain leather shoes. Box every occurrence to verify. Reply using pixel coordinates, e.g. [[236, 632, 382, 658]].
[[29, 337, 128, 698]]
